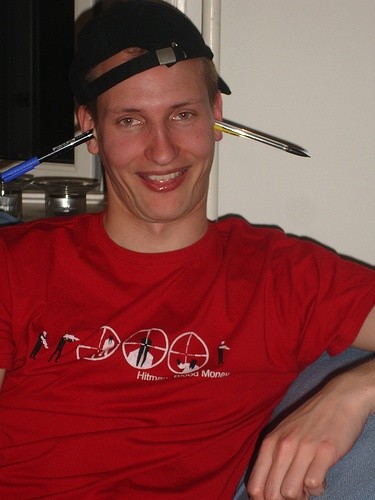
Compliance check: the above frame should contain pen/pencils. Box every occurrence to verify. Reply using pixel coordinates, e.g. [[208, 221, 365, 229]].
[[0, 128, 95, 183], [213, 120, 312, 158]]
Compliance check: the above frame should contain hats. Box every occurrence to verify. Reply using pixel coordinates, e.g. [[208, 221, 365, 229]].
[[69, 0, 231, 104]]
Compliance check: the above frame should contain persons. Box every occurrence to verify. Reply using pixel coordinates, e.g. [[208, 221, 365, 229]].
[[0, 0, 375, 500]]
